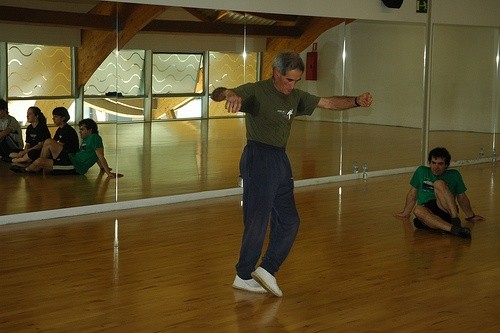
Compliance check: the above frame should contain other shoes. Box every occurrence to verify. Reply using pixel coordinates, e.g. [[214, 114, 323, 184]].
[[232, 275, 268, 293], [10, 165, 26, 173], [29, 169, 43, 175], [450, 225, 471, 240], [251, 266, 282, 297], [449, 217, 461, 229], [0, 157, 13, 163]]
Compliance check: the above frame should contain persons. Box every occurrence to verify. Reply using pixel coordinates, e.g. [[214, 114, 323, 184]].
[[395, 146, 484, 238], [0, 98, 123, 179], [211, 50, 374, 297]]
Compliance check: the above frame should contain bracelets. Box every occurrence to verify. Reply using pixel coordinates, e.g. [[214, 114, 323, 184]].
[[468, 215, 475, 219], [355, 97, 361, 106]]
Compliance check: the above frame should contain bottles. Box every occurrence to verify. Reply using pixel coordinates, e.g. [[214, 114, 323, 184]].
[[362, 162, 368, 182], [352, 161, 359, 174], [492, 148, 497, 163], [479, 148, 485, 158]]
